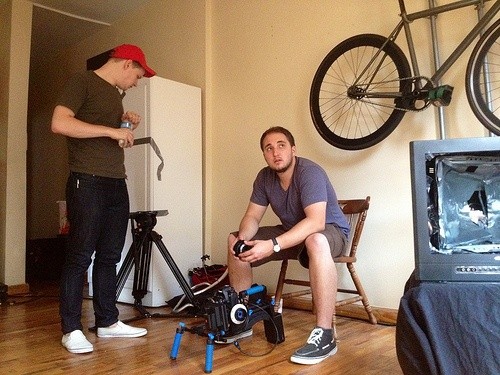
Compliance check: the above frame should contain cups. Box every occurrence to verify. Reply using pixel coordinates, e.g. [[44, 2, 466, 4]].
[[271, 296, 283, 314]]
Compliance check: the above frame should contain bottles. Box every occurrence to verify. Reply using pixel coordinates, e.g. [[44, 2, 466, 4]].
[[118, 120, 133, 147]]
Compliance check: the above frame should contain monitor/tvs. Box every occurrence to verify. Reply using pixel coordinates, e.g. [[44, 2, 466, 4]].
[[409, 136, 500, 282]]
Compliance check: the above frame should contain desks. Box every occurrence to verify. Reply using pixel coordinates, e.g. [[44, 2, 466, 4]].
[[395, 269, 500, 375]]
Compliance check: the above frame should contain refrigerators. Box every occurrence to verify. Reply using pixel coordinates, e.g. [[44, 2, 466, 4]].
[[88, 74, 204, 307]]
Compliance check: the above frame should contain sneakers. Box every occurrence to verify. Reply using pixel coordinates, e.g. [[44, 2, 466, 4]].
[[61, 329, 94, 353], [214, 322, 253, 344], [97, 319, 148, 338], [291, 325, 337, 365]]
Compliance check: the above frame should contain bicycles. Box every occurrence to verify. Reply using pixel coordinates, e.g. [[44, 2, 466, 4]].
[[308, 0, 500, 153]]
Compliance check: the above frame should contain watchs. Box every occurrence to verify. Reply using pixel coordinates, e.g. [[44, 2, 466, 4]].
[[271, 237, 281, 254]]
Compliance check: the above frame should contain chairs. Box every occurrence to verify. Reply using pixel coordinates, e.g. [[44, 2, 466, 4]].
[[274, 196, 378, 323]]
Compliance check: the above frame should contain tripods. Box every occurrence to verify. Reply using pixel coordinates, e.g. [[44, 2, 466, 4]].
[[116, 210, 205, 316]]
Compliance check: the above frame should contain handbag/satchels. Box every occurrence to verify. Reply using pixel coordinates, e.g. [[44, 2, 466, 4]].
[[189, 263, 229, 289]]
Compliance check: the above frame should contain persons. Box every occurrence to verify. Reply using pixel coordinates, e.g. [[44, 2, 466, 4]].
[[214, 126, 352, 365], [50, 44, 156, 354]]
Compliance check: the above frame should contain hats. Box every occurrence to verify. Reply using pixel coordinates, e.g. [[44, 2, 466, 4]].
[[110, 44, 157, 78]]
